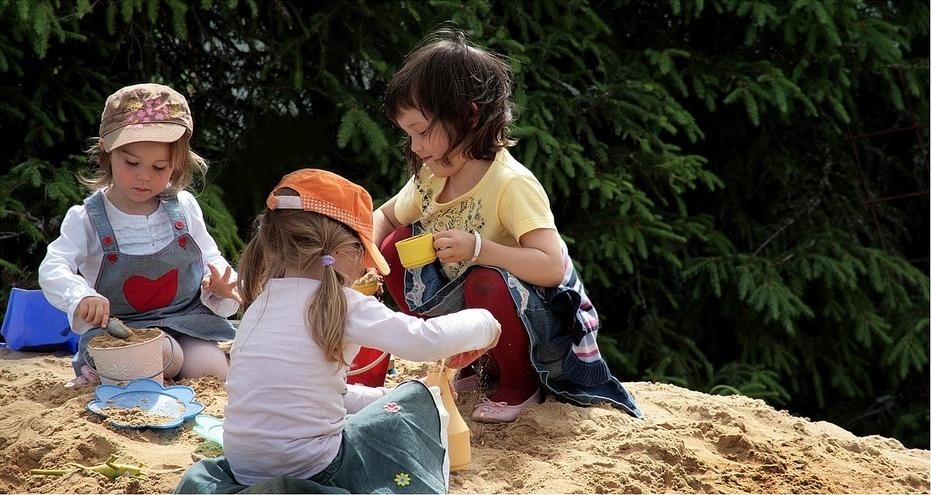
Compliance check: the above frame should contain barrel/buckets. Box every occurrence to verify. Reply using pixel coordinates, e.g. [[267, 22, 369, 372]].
[[83, 328, 174, 387], [344, 346, 391, 387]]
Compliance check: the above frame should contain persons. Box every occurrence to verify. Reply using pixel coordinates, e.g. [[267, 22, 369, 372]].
[[39, 81, 244, 383], [373, 18, 643, 427], [219, 166, 504, 494]]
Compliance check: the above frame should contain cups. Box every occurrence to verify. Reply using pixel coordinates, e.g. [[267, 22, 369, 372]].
[[394, 233, 438, 270]]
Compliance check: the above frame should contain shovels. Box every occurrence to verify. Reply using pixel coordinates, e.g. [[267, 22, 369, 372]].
[[2, 287, 82, 355]]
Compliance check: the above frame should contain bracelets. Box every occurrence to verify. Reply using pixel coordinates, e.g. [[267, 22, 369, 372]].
[[470, 229, 483, 263]]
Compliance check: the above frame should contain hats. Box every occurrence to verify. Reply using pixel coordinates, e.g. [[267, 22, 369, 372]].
[[267, 168, 393, 277], [98, 81, 194, 154]]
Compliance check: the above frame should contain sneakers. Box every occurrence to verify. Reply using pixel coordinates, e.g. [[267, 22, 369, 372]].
[[472, 384, 543, 423]]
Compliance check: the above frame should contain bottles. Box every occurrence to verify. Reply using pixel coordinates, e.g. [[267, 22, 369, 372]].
[[424, 362, 472, 468]]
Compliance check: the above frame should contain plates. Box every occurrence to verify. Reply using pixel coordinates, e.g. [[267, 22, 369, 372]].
[[193, 415, 224, 446], [88, 377, 206, 431]]
